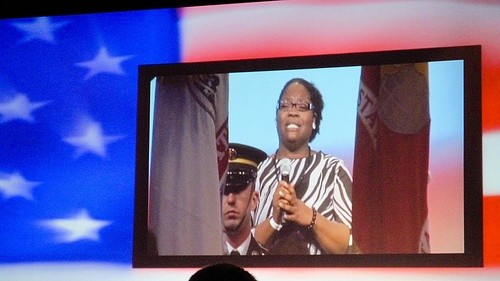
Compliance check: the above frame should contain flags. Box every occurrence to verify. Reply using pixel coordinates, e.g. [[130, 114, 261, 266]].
[[350, 60, 432, 255], [148, 73, 230, 255]]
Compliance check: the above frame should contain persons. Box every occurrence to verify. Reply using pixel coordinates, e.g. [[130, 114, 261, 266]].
[[189, 263, 256, 281], [222, 143, 267, 256], [251, 78, 355, 254]]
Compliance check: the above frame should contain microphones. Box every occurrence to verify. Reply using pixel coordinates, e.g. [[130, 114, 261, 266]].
[[280, 156, 291, 211]]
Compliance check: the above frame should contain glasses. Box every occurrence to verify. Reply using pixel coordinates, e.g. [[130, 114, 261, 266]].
[[223, 183, 250, 196], [279, 102, 315, 111]]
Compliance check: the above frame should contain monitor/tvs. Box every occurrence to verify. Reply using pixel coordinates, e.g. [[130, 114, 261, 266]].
[[131, 45, 480, 268]]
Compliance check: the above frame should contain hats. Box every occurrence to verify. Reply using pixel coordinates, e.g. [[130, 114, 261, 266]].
[[226, 143, 267, 182]]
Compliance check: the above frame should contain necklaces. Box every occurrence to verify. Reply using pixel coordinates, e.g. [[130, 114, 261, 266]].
[[274, 146, 311, 188]]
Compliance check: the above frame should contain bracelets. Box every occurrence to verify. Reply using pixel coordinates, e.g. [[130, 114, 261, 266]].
[[304, 207, 317, 231], [270, 217, 282, 231]]
